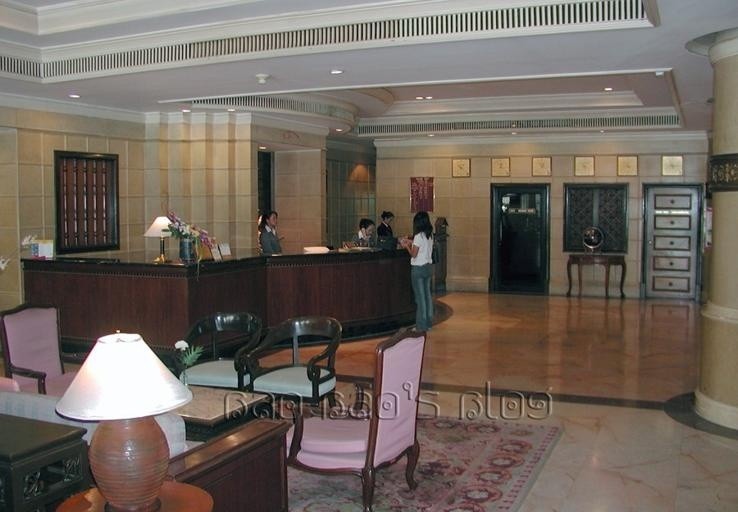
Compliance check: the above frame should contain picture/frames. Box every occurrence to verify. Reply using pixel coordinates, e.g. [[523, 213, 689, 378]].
[[410, 177, 434, 213]]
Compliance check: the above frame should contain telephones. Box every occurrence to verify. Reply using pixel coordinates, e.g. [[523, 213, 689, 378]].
[[361, 227, 369, 239]]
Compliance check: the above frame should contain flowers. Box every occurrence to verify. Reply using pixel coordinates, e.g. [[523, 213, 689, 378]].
[[167, 212, 215, 282], [168, 338, 203, 379]]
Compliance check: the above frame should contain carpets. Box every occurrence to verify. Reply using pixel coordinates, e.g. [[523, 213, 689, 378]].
[[266, 407, 564, 512]]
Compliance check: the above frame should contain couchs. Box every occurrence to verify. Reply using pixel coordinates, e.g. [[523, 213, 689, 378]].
[[0, 376, 205, 460]]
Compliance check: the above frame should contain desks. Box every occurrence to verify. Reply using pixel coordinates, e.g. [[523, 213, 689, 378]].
[[567, 254, 625, 299]]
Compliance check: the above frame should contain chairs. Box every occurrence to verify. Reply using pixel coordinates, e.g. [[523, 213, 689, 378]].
[[241, 315, 342, 407], [285, 325, 427, 512], [176, 313, 264, 391], [0, 302, 79, 396]]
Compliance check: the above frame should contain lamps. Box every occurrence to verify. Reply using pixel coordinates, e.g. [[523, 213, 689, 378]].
[[55, 334, 214, 512], [255, 74, 269, 86], [141, 216, 174, 264]]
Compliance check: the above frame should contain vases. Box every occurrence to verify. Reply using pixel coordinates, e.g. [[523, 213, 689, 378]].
[[179, 238, 196, 262], [179, 375, 194, 399]]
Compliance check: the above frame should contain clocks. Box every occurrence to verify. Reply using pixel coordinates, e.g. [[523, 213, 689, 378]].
[[532, 157, 551, 177], [490, 158, 510, 177], [451, 157, 471, 178], [575, 155, 594, 176], [661, 155, 683, 176], [617, 155, 638, 176]]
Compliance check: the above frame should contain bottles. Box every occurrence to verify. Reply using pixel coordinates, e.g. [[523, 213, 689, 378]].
[[360, 228, 368, 247]]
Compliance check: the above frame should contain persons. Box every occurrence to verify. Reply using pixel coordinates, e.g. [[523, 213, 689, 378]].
[[260, 210, 282, 254], [400, 210, 435, 331], [351, 218, 378, 247], [376, 211, 395, 239]]
